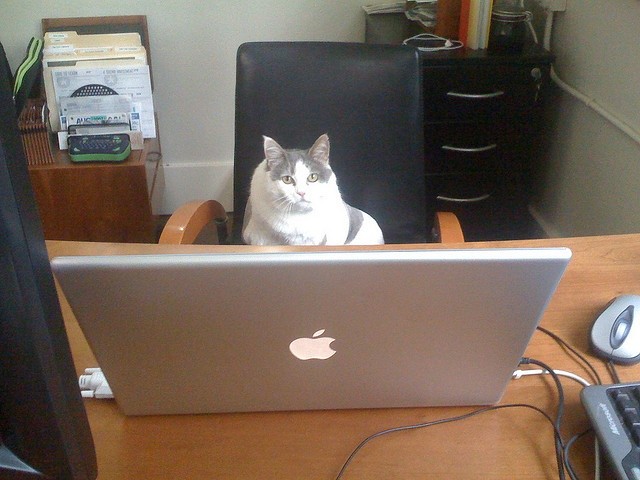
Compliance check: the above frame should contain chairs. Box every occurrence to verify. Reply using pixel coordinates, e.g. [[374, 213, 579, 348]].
[[159, 42, 464, 244]]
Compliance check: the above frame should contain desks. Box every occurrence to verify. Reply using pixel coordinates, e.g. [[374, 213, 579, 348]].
[[44, 233, 640, 480]]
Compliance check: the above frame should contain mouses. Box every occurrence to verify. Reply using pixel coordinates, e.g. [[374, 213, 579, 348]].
[[587, 295, 640, 365]]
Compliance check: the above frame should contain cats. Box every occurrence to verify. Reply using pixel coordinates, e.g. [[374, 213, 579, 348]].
[[241, 132, 385, 246]]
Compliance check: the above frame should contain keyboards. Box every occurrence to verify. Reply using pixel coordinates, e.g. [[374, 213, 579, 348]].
[[579, 382, 640, 480]]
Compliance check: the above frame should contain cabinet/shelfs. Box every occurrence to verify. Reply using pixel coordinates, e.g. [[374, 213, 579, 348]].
[[365, 13, 555, 241], [18, 97, 166, 243]]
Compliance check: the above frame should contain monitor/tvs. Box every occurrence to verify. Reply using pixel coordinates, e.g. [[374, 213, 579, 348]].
[[1, 63, 98, 479]]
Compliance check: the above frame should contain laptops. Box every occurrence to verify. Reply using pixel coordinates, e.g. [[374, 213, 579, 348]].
[[50, 245, 573, 416]]
